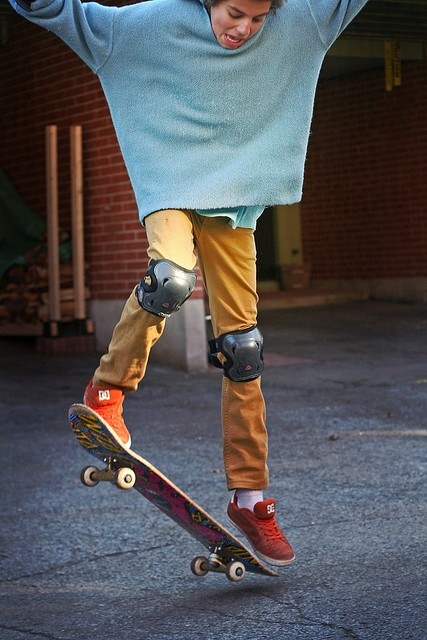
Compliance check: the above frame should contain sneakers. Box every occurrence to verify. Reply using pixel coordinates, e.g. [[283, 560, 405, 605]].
[[83, 372, 132, 451], [227, 490, 296, 566]]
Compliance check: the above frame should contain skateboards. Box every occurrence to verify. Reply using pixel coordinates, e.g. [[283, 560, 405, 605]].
[[68, 404, 278, 581]]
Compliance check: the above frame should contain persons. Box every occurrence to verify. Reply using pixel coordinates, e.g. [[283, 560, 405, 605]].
[[7, 0, 369, 566]]
